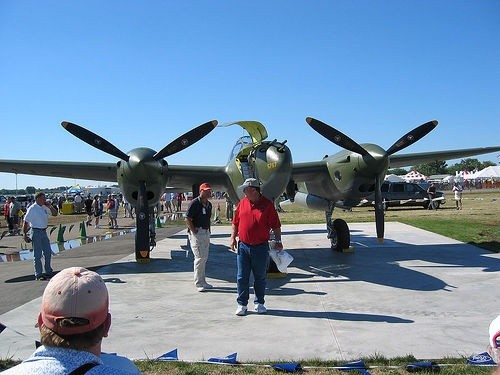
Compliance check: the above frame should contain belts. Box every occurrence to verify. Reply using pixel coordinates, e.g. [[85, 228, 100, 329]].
[[32, 228, 46, 231], [196, 226, 209, 229]]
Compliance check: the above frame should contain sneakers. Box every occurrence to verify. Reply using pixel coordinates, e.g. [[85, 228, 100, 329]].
[[255, 303, 267, 313], [236, 305, 248, 315]]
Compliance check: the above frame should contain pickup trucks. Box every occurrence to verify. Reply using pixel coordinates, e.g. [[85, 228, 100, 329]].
[[365, 182, 441, 210]]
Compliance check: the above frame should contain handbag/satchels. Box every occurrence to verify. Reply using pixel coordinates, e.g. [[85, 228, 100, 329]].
[[18, 210, 24, 218]]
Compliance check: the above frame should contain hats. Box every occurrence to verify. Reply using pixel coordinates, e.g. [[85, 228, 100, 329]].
[[41, 267, 108, 334], [489, 315, 500, 349], [11, 197, 16, 200], [200, 183, 212, 191], [240, 178, 260, 189]]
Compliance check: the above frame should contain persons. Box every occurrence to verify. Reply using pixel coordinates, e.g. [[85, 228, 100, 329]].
[[0, 267, 142, 375], [184, 183, 213, 292], [22, 193, 58, 281], [4, 191, 233, 237], [452, 182, 463, 210], [426, 183, 437, 211], [229, 178, 283, 316], [488, 314, 500, 375]]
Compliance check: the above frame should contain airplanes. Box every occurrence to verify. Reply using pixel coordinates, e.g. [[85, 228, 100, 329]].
[[0, 116, 500, 263]]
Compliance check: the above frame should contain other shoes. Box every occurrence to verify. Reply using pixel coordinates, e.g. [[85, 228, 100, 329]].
[[203, 283, 212, 289], [95, 226, 101, 229], [110, 225, 118, 229], [197, 287, 204, 291], [8, 233, 15, 236], [89, 224, 91, 225], [45, 274, 55, 277], [36, 275, 45, 281], [86, 222, 88, 227], [14, 229, 21, 234]]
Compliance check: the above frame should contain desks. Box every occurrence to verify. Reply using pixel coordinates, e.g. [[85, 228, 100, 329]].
[[62, 202, 75, 214]]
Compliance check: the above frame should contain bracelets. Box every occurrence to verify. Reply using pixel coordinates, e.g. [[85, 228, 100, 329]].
[[49, 205, 53, 209], [274, 240, 282, 244]]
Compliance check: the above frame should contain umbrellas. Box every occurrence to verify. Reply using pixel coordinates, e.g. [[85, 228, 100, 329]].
[[64, 185, 88, 194]]
